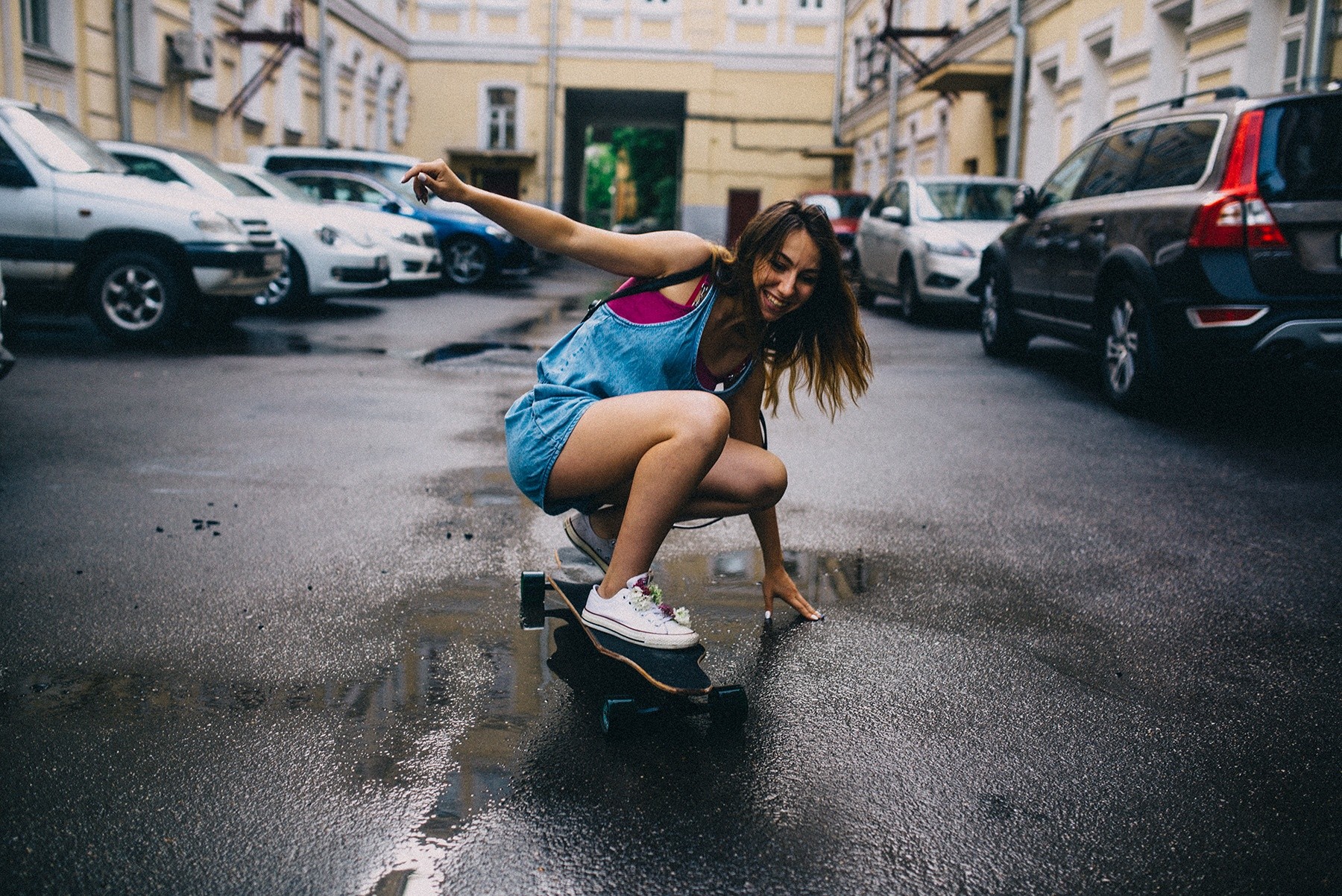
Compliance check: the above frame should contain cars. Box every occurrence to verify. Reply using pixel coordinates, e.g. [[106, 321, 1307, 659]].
[[92, 134, 392, 319], [797, 190, 874, 273], [242, 165, 443, 293], [280, 169, 531, 289], [852, 174, 1037, 322]]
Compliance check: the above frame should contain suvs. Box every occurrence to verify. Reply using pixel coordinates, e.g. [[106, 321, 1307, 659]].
[[978, 73, 1342, 413], [253, 143, 529, 265], [1, 99, 291, 354]]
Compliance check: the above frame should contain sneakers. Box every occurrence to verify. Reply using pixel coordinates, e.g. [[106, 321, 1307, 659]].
[[562, 510, 618, 578], [580, 572, 700, 651]]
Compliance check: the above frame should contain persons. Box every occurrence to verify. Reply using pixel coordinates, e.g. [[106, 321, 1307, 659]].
[[401, 159, 877, 652]]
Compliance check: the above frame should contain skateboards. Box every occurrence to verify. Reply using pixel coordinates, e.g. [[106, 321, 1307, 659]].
[[519, 566, 752, 740]]
